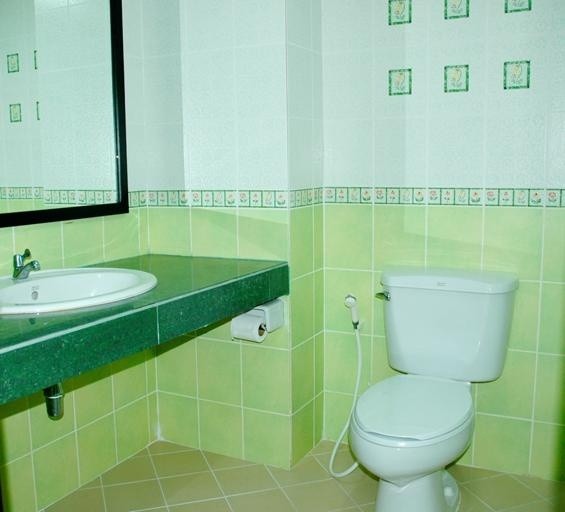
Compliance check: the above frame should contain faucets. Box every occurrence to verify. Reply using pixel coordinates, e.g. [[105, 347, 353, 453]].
[[13, 249, 40, 279]]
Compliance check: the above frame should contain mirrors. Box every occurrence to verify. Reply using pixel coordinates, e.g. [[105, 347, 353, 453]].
[[0, 0, 130, 233]]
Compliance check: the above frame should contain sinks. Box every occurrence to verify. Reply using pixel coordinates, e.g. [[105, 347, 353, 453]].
[[0, 267, 158, 314]]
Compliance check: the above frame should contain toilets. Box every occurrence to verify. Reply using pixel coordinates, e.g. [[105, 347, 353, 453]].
[[348, 266, 518, 512]]
